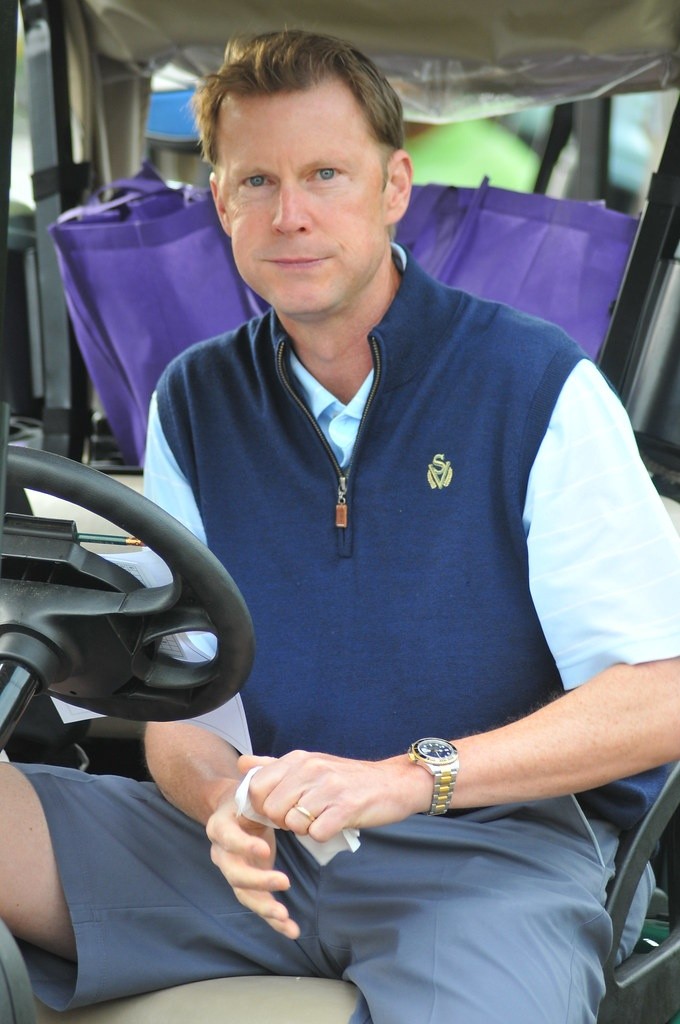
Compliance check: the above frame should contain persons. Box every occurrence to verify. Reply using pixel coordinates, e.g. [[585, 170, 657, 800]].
[[0, 30, 680, 1024]]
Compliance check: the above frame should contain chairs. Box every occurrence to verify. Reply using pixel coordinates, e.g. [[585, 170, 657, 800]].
[[17, 430, 680, 1024]]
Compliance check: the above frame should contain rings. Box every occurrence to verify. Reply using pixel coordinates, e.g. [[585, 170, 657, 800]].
[[293, 804, 317, 823]]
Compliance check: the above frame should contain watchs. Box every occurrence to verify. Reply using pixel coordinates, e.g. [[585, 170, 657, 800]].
[[407, 737, 459, 818]]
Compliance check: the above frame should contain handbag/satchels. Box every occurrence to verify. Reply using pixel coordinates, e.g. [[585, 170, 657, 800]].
[[48, 162, 272, 472], [393, 177, 639, 361]]
